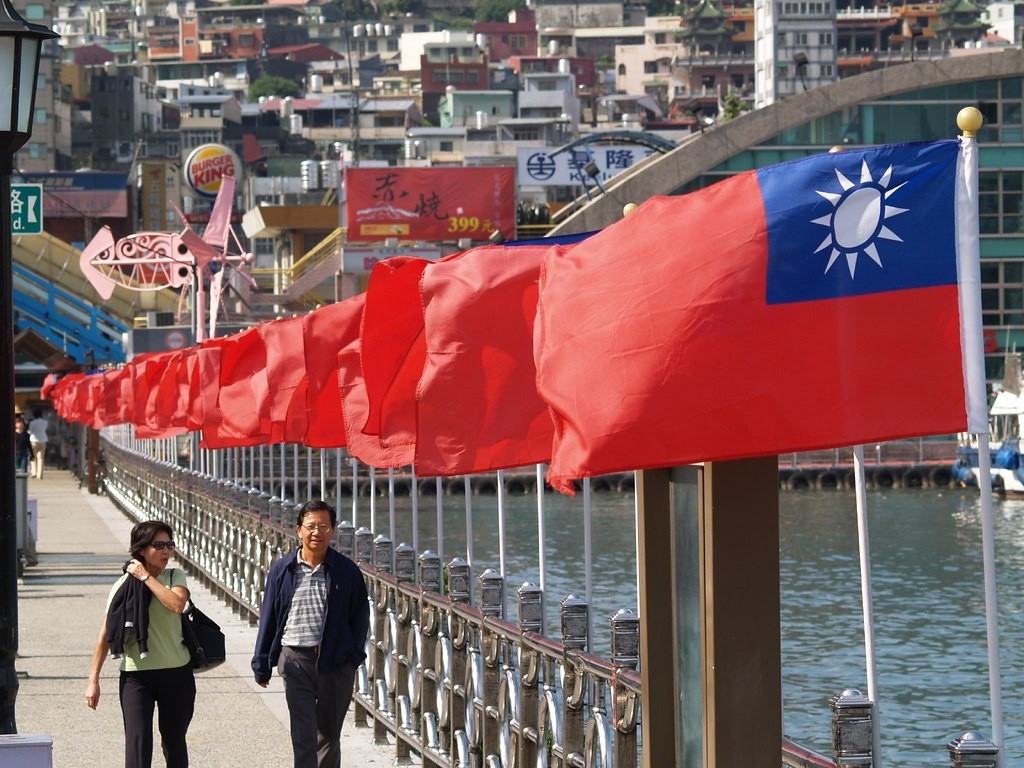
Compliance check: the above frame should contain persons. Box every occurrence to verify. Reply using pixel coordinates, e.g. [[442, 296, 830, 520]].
[[85, 521, 196, 768], [250, 500, 370, 768], [27, 409, 48, 480], [15, 404, 34, 473]]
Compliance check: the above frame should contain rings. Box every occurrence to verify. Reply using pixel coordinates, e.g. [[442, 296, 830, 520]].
[[85, 701, 88, 703]]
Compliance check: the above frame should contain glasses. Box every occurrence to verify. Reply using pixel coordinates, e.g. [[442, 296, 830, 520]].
[[149, 541, 175, 551], [301, 524, 332, 533]]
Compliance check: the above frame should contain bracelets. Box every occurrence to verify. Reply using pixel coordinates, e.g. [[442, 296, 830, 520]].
[[142, 574, 150, 581]]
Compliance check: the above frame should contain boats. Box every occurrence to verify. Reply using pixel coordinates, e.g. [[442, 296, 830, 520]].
[[951, 342, 1024, 491]]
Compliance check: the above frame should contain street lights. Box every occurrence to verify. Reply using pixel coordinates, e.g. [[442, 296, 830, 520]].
[[0, 0, 63, 734]]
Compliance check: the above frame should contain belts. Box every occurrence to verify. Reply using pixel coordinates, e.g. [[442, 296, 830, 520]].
[[289, 645, 318, 654]]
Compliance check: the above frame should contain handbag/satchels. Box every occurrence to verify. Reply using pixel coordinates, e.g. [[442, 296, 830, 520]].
[[168, 568, 226, 675]]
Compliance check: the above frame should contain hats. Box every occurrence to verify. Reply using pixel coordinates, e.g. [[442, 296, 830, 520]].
[[15, 402, 25, 414]]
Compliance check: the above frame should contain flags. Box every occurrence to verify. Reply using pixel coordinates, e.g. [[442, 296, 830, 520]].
[[360, 256, 436, 449], [50, 317, 306, 460], [545, 139, 990, 494], [284, 291, 376, 448], [339, 340, 416, 470], [413, 246, 556, 478]]
